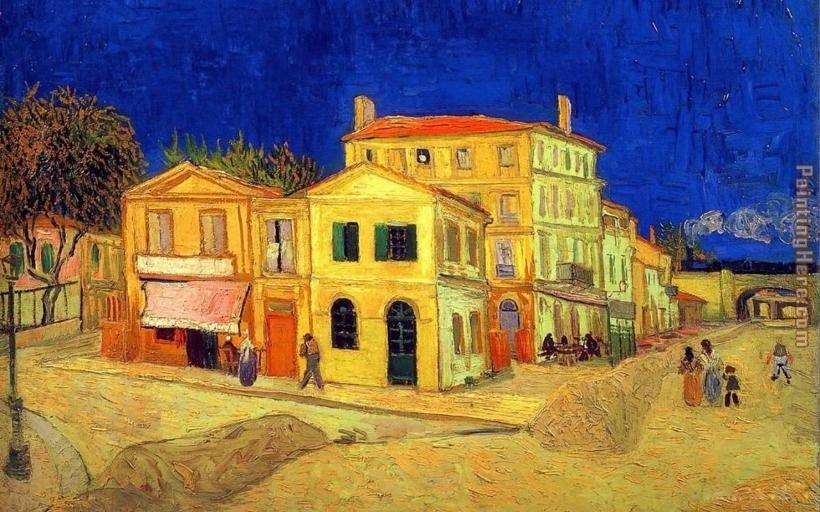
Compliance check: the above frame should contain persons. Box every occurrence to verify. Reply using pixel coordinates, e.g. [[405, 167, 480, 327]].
[[676, 340, 740, 411], [580, 333, 601, 361], [222, 336, 240, 376], [298, 332, 327, 393], [770, 338, 793, 385], [544, 333, 555, 358]]
